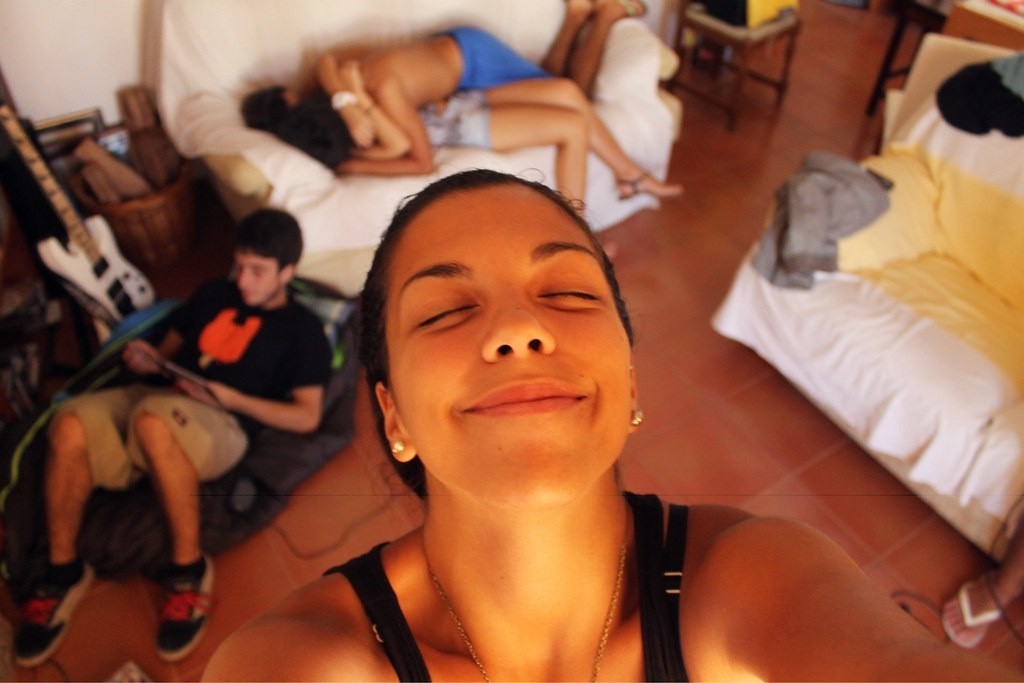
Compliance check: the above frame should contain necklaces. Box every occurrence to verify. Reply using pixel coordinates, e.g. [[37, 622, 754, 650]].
[[422, 545, 628, 683]]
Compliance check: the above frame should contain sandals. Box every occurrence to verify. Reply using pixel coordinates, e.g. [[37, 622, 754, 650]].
[[618, 174, 683, 202]]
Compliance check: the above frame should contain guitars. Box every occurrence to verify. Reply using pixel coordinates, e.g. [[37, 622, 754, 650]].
[[0, 96, 156, 348]]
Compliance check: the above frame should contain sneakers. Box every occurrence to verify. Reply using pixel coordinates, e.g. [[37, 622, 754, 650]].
[[156, 554, 213, 661], [15, 558, 95, 668]]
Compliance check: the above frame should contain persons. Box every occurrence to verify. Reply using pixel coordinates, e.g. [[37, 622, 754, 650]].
[[240, 0, 683, 264], [201, 169, 1024, 683], [12, 208, 333, 666]]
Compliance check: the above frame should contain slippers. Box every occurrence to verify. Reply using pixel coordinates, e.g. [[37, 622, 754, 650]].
[[621, 0, 649, 17], [943, 580, 1001, 648]]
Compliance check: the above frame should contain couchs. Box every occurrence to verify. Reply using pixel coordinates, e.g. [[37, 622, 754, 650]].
[[144, 0, 686, 305], [709, 51, 1024, 568]]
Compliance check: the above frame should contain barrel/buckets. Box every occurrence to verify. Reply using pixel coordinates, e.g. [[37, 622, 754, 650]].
[[69, 127, 192, 269]]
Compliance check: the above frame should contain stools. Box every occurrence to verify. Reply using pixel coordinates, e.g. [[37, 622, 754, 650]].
[[677, 2, 803, 128]]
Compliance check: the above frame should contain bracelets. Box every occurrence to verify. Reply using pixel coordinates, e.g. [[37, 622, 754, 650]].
[[363, 101, 376, 114], [331, 91, 357, 110]]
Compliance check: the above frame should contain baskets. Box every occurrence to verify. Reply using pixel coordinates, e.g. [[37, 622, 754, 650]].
[[71, 122, 201, 267]]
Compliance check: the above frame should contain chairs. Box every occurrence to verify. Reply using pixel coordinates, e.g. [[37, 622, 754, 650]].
[[874, 32, 1022, 157]]
